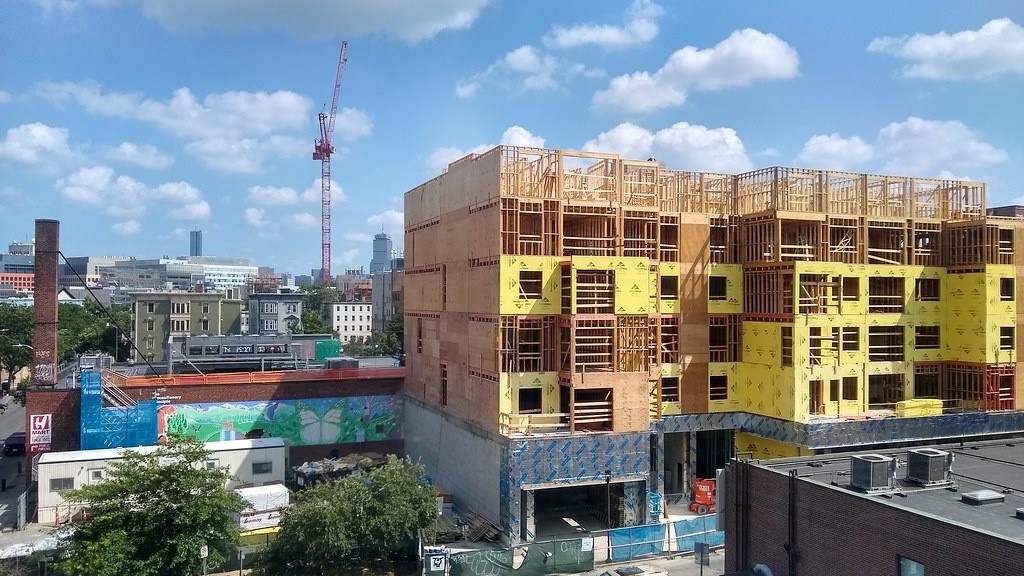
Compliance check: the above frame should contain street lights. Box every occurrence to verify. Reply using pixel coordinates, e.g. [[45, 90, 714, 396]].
[[106, 322, 118, 361], [604, 470, 613, 562]]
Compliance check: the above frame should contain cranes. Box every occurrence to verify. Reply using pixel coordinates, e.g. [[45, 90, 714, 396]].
[[310, 40, 351, 285]]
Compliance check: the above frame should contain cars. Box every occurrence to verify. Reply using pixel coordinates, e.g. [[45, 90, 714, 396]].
[[3, 431, 25, 457]]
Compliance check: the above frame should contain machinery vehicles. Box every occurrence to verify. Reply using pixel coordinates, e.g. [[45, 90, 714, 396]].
[[688, 475, 718, 515]]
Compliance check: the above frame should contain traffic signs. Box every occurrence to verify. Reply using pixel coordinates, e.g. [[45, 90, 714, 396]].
[[30, 413, 53, 454]]
